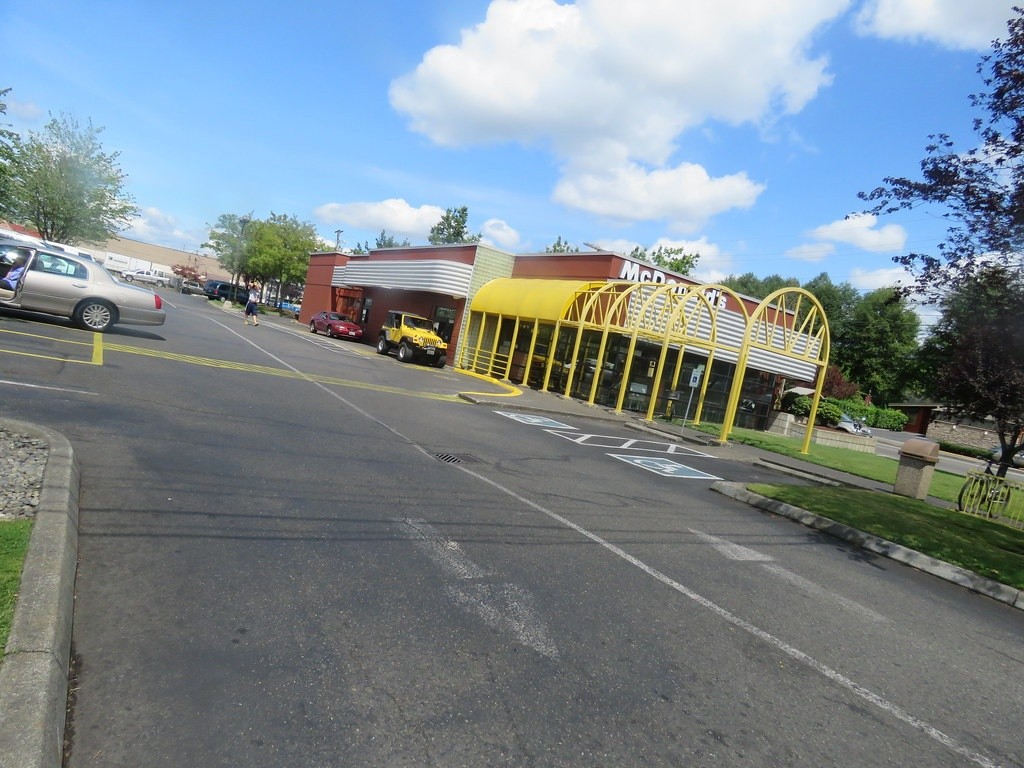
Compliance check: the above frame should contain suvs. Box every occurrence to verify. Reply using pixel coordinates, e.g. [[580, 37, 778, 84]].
[[377, 309, 448, 368], [201, 279, 249, 305], [180, 280, 205, 295]]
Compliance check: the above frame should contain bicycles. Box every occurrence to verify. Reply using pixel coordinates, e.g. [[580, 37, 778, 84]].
[[959, 455, 1014, 519]]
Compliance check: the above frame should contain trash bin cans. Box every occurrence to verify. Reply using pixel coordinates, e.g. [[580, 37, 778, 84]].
[[893, 438, 940, 499]]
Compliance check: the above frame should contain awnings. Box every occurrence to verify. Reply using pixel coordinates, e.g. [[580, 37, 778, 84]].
[[330, 266, 352, 289], [343, 260, 473, 297]]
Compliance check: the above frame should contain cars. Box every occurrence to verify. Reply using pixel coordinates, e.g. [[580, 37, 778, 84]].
[[308, 310, 363, 343], [827, 410, 872, 438], [564, 357, 615, 382], [987, 444, 1024, 470], [0, 226, 167, 334], [256, 291, 303, 311]]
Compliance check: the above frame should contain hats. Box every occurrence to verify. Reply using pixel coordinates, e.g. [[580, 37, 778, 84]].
[[12, 257, 25, 265]]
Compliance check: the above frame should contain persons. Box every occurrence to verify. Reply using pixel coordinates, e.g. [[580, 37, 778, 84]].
[[0, 257, 26, 290], [244, 283, 260, 326]]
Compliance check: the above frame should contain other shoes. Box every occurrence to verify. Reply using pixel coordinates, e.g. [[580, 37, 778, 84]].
[[244, 322, 248, 324], [255, 323, 258, 326]]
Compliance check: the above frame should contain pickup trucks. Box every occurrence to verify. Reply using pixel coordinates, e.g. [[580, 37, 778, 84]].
[[120, 269, 171, 287]]
